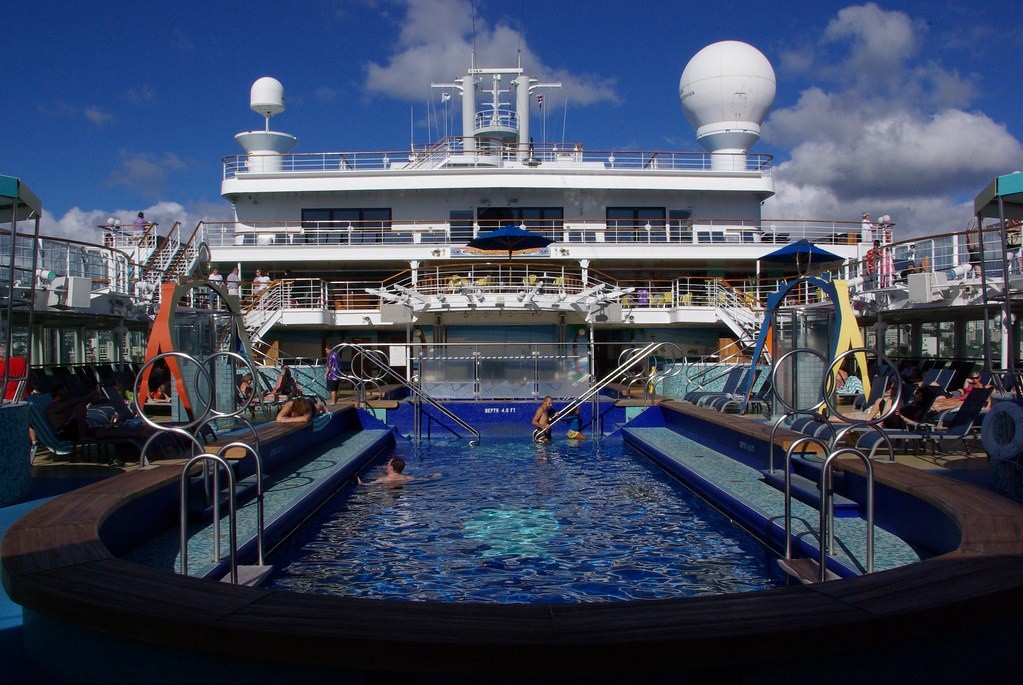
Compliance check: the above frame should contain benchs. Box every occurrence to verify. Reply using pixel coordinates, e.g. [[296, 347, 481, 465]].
[[0, 459, 1023, 685], [597, 383, 826, 472], [203, 384, 407, 489]]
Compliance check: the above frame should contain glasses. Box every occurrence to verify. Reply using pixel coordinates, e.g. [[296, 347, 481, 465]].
[[915, 392, 920, 395], [971, 377, 980, 379], [886, 386, 896, 390]]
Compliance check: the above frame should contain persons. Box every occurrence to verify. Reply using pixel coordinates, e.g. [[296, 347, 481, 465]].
[[226, 266, 242, 295], [252, 269, 263, 294], [283, 270, 295, 308], [259, 270, 271, 311], [272, 365, 303, 398], [865, 212, 1022, 288], [323, 344, 341, 407], [50, 380, 174, 411], [827, 359, 1022, 430], [275, 397, 329, 423], [532, 395, 560, 447], [134, 211, 148, 247], [355, 455, 442, 486], [239, 372, 276, 402], [208, 267, 223, 311]]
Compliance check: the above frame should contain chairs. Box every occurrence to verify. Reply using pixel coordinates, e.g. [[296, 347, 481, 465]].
[[683, 358, 1022, 459], [25, 363, 327, 466], [444, 274, 755, 308]]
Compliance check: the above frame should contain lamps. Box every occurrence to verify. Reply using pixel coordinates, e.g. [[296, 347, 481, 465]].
[[364, 284, 634, 308]]
